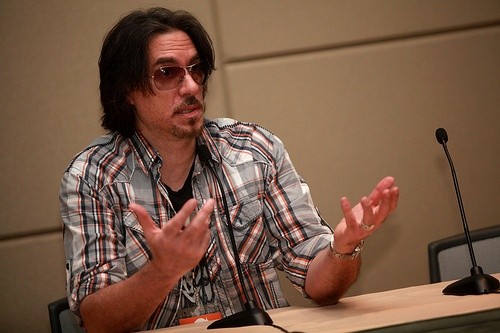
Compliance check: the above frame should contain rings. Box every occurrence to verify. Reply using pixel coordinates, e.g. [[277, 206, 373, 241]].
[[361, 221, 375, 230]]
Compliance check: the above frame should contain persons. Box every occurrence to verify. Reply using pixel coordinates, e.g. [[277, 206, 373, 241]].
[[58, 8, 399, 333]]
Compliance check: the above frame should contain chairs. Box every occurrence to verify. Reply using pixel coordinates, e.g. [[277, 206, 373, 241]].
[[428, 224, 500, 283]]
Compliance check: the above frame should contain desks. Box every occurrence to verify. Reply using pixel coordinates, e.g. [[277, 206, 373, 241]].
[[137, 273, 500, 332]]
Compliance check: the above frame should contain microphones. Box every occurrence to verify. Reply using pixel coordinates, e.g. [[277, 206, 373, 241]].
[[435, 128, 500, 296], [196, 144, 274, 330]]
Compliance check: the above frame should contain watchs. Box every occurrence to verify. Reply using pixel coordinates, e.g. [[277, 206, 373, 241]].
[[328, 233, 365, 260]]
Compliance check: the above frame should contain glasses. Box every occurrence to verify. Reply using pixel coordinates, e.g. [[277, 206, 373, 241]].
[[146, 62, 207, 91]]
